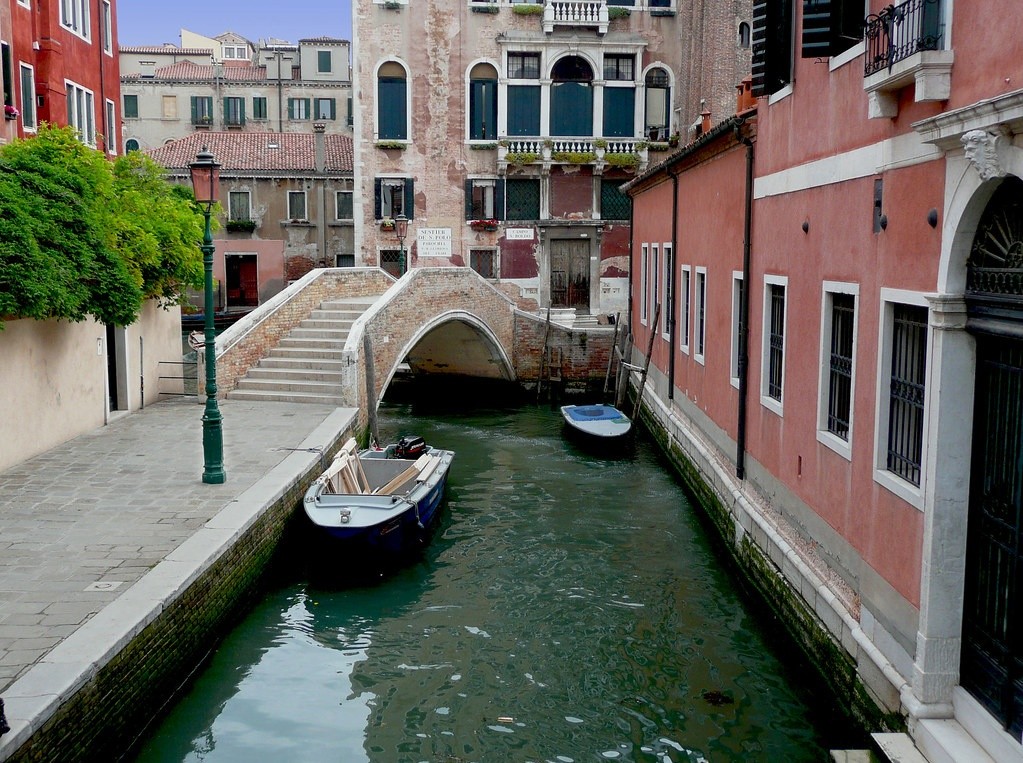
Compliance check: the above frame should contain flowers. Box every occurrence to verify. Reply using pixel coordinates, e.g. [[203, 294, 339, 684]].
[[469, 219, 500, 232]]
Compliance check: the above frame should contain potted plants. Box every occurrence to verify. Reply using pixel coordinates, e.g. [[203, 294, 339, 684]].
[[668, 134, 680, 148], [224, 216, 256, 233], [381, 220, 397, 231]]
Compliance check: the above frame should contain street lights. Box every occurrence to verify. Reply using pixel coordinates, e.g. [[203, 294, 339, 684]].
[[187, 145, 229, 487]]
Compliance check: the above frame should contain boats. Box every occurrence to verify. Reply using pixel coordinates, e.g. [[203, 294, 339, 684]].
[[561, 403, 632, 439], [304, 432, 457, 555]]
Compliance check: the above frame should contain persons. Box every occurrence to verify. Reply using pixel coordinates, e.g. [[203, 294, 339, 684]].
[[961, 129, 999, 180]]
